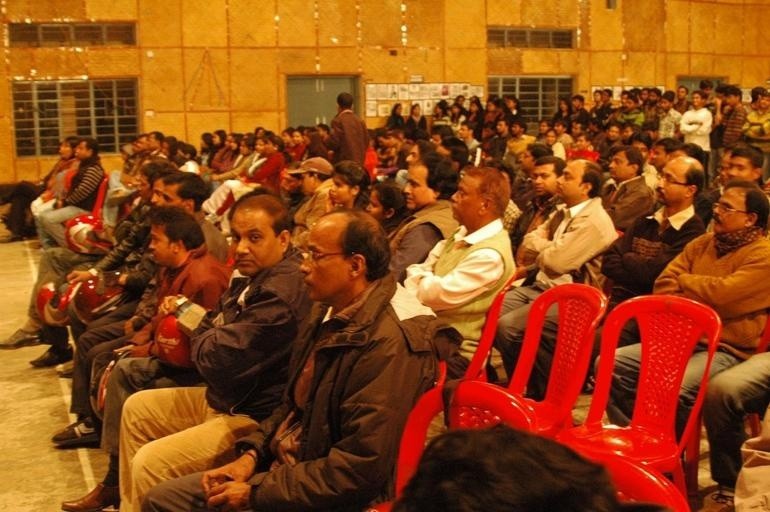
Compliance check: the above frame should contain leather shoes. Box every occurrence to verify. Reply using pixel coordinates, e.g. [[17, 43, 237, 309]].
[[56, 366, 76, 379], [0, 327, 43, 351], [29, 343, 75, 369], [59, 480, 121, 512]]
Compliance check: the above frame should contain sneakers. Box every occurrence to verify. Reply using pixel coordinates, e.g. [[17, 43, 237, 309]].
[[51, 414, 102, 449], [697, 490, 736, 512]]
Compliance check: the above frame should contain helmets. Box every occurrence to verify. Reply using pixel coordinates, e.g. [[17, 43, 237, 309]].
[[71, 272, 132, 325], [154, 311, 195, 369], [34, 278, 80, 328], [63, 213, 115, 258]]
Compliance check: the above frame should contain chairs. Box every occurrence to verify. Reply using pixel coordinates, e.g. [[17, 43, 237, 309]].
[[37, 172, 197, 419], [372, 274, 721, 512], [565, 147, 600, 162], [744, 321, 768, 438]]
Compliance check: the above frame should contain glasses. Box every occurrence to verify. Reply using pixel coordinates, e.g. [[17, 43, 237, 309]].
[[656, 169, 691, 186], [307, 245, 355, 261], [710, 201, 753, 215]]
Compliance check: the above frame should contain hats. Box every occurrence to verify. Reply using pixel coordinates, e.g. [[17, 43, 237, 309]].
[[286, 155, 336, 178], [336, 91, 354, 109]]
